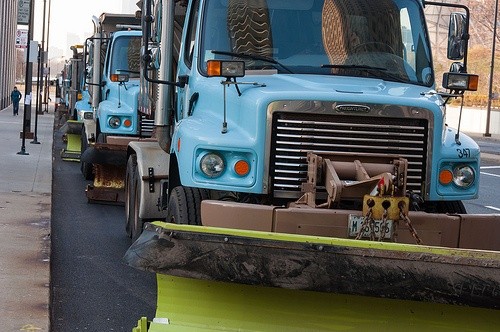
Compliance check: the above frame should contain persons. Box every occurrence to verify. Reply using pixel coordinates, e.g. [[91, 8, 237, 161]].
[[11, 87, 22, 116]]
